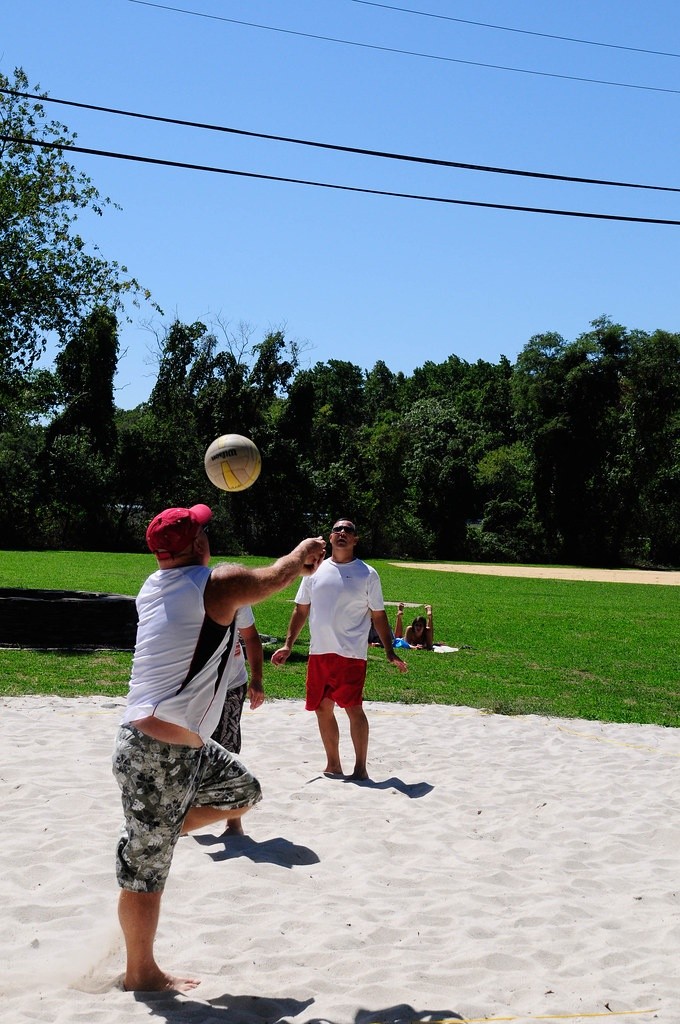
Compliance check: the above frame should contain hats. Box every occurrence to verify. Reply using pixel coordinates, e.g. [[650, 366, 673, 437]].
[[148, 503, 211, 559]]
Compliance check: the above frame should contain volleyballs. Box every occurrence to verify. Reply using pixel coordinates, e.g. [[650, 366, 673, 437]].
[[203, 432, 263, 494]]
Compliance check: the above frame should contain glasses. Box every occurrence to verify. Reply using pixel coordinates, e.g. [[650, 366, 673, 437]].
[[332, 526, 357, 537]]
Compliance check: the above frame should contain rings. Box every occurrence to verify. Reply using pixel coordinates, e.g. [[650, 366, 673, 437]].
[[261, 699, 263, 702]]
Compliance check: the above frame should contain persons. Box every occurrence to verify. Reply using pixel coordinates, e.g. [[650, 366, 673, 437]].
[[366, 613, 394, 647], [209, 561, 265, 837], [113, 501, 326, 994], [271, 520, 409, 777], [394, 602, 434, 652]]
